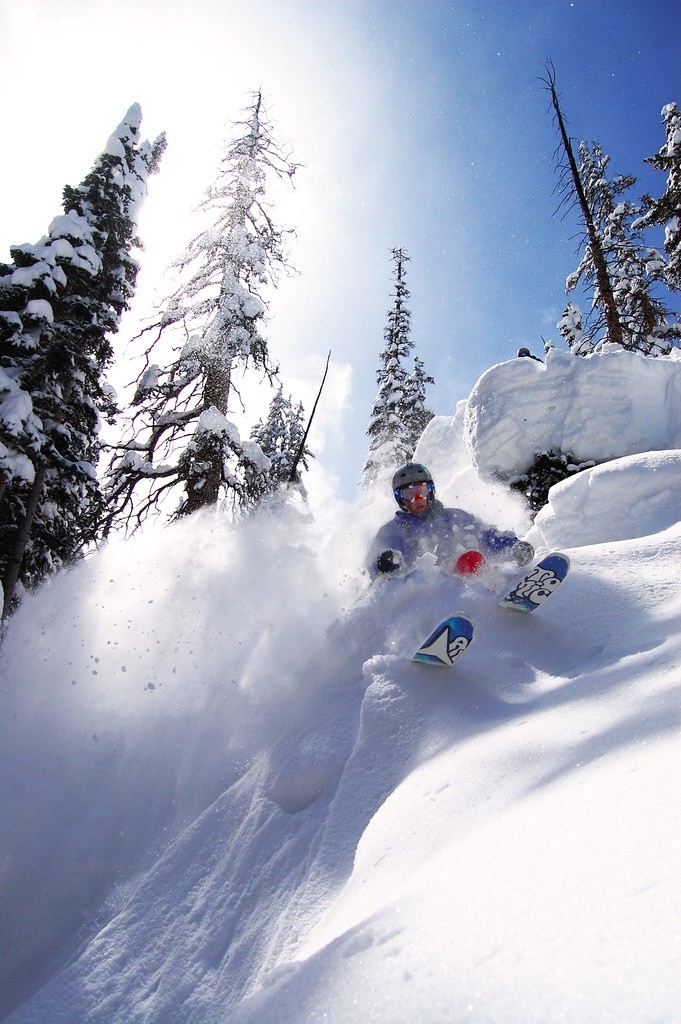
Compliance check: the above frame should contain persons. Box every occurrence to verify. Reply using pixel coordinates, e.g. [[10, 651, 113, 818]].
[[364, 463, 535, 575], [518, 348, 544, 363]]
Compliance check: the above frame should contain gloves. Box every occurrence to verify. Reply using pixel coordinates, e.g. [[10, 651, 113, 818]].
[[510, 540, 535, 567], [376, 549, 400, 573]]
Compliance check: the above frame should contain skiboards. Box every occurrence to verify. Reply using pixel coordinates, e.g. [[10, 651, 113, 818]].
[[410, 551, 571, 667]]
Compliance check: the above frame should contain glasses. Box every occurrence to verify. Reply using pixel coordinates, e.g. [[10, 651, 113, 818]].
[[400, 484, 432, 502]]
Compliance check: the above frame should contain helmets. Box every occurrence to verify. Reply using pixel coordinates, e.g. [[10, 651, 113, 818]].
[[391, 462, 434, 491]]
